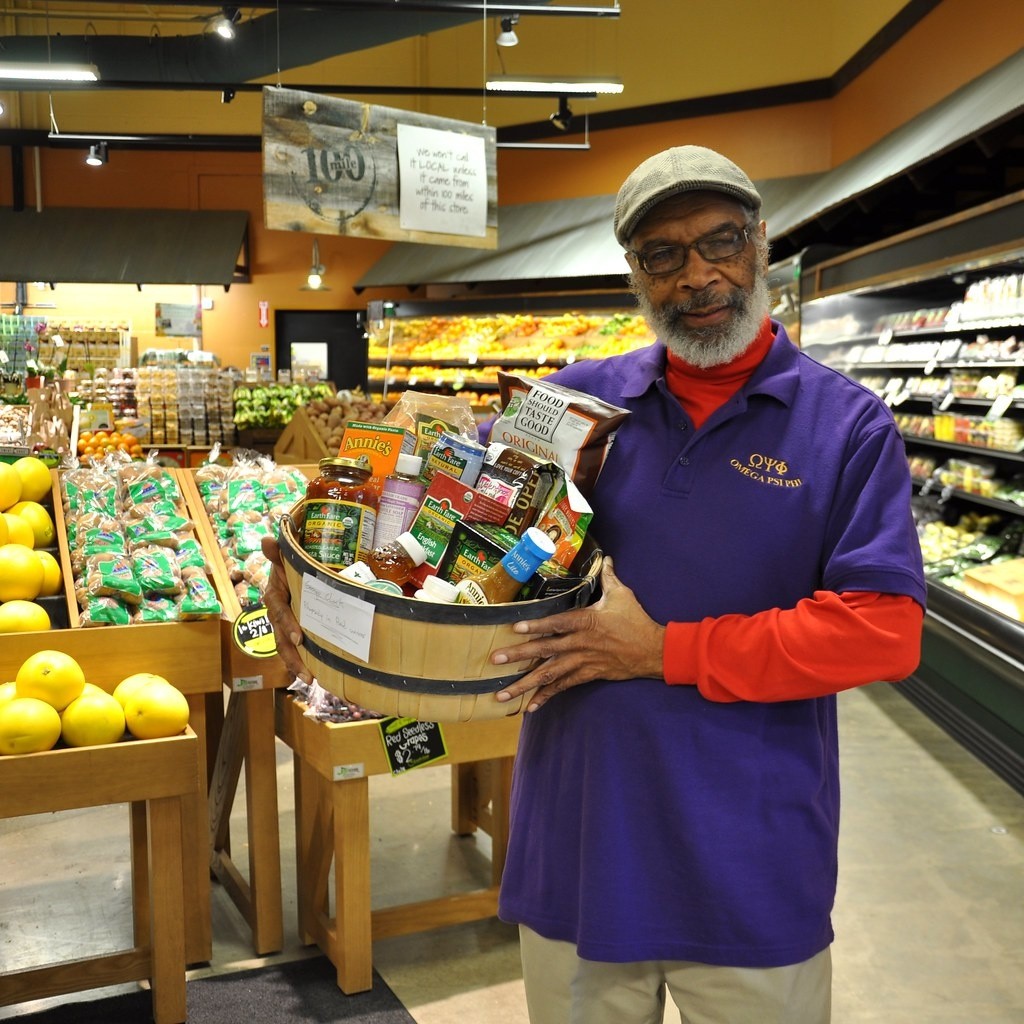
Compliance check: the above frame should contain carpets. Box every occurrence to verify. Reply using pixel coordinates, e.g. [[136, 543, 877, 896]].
[[1, 956, 419, 1024]]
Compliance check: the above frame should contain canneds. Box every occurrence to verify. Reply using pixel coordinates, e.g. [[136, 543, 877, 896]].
[[297, 456, 379, 572]]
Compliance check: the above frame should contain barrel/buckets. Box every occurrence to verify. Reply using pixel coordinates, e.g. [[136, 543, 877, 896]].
[[280, 500, 599, 721]]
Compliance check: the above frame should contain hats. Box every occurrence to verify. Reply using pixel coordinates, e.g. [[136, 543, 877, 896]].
[[613, 144, 762, 247]]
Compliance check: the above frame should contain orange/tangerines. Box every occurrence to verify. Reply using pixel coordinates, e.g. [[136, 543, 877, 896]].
[[368, 312, 654, 361], [78, 431, 144, 464], [369, 365, 560, 406]]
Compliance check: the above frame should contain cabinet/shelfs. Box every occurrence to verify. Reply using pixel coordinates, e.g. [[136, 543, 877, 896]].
[[36, 317, 133, 372], [352, 48, 1024, 795]]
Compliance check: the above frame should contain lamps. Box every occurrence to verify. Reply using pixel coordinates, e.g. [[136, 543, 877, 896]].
[[217, 6, 241, 41], [0, 78, 597, 151], [486, 74, 624, 95], [0, 0, 101, 83], [85, 145, 104, 165], [549, 96, 573, 132], [68, 0, 621, 18], [495, 11, 521, 46], [307, 239, 325, 289]]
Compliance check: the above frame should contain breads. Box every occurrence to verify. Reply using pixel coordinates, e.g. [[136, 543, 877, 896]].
[[195, 463, 310, 605], [60, 464, 223, 627]]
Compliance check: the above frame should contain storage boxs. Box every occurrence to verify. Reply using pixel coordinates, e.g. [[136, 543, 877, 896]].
[[437, 519, 584, 602], [404, 471, 512, 589], [336, 420, 421, 513]]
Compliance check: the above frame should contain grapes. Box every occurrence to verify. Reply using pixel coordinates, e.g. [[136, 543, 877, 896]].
[[314, 690, 382, 722]]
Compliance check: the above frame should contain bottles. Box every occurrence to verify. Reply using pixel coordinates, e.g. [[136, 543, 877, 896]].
[[412, 575, 459, 604], [336, 532, 426, 587], [456, 529, 557, 604], [373, 452, 426, 547]]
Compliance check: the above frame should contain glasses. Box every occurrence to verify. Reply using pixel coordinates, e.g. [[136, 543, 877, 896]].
[[633, 222, 757, 276]]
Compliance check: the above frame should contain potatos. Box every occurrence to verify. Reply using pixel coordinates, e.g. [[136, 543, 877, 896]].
[[308, 395, 387, 456]]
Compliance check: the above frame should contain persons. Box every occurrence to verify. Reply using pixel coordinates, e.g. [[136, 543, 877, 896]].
[[261, 145, 926, 1023]]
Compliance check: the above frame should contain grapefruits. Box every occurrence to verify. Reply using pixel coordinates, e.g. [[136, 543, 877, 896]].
[[0, 456, 63, 634], [0, 649, 189, 755]]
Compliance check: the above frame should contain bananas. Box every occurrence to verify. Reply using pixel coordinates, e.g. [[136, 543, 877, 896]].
[[232, 383, 334, 432]]
